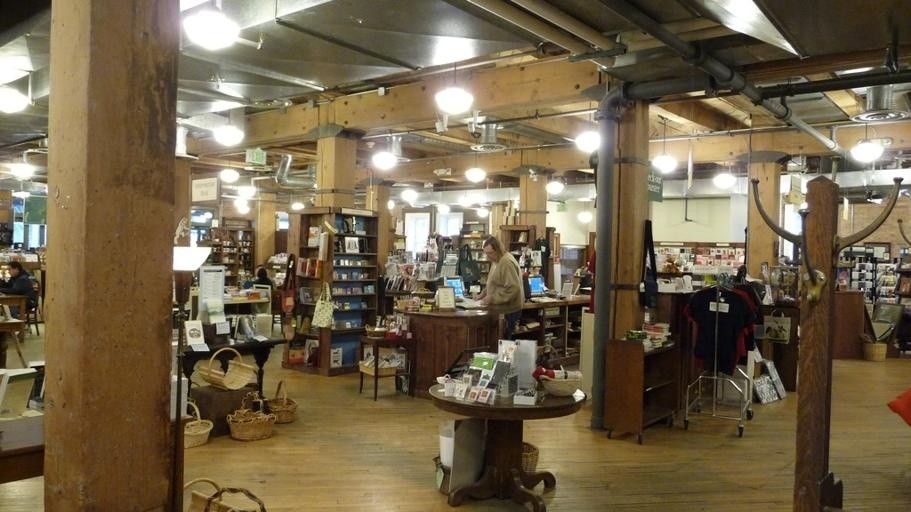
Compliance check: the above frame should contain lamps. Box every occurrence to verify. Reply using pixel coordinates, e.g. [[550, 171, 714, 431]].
[[173, 246, 212, 512], [850, 124, 886, 164]]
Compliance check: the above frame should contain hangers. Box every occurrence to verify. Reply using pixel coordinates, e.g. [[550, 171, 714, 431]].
[[710, 271, 749, 291]]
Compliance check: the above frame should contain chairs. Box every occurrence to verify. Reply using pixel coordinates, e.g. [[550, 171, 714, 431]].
[[271, 291, 283, 335], [25, 279, 42, 336]]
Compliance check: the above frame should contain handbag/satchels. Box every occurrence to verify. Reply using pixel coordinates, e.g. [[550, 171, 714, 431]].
[[535, 236, 551, 259], [281, 255, 296, 313], [639, 219, 658, 309], [459, 244, 481, 283], [762, 309, 792, 345], [311, 281, 334, 329]]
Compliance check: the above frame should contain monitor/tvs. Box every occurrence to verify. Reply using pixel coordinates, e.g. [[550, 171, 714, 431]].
[[444, 276, 465, 297], [528, 275, 545, 297]]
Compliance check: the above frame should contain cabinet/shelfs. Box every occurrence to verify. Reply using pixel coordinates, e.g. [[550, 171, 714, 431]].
[[656, 283, 801, 392], [769, 235, 911, 359], [384, 220, 591, 398], [602, 335, 679, 443], [648, 238, 746, 276], [0, 241, 39, 291], [197, 215, 255, 285], [283, 206, 379, 378]]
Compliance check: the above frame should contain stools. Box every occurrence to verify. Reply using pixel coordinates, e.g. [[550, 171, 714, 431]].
[[187, 383, 260, 438]]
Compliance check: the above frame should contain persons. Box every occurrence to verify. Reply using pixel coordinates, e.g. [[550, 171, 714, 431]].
[[475, 237, 525, 341], [252, 268, 274, 290], [1, 260, 36, 318]]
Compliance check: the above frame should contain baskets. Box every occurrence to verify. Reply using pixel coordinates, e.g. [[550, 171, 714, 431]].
[[268, 379, 297, 424], [521, 442, 539, 473], [540, 375, 581, 397], [225, 392, 277, 440], [184, 401, 214, 449], [359, 361, 396, 377], [861, 341, 888, 362]]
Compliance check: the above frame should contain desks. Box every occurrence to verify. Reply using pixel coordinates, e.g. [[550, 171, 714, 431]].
[[0, 295, 27, 342], [188, 284, 274, 334], [358, 334, 417, 400], [177, 334, 288, 414], [428, 376, 585, 511]]
